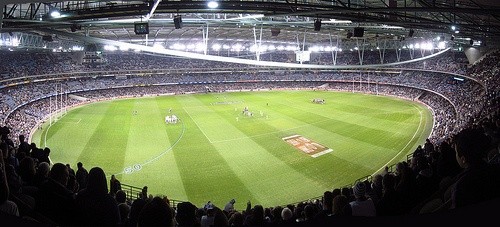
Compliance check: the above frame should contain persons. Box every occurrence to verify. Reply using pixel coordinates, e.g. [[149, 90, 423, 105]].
[[1, 44, 500, 227]]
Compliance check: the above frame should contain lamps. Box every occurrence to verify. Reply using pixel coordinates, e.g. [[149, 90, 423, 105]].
[[346, 33, 353, 39], [405, 29, 415, 37], [70, 23, 77, 33], [173, 8, 183, 30], [271, 28, 280, 36], [134, 14, 149, 34], [313, 19, 323, 30]]
